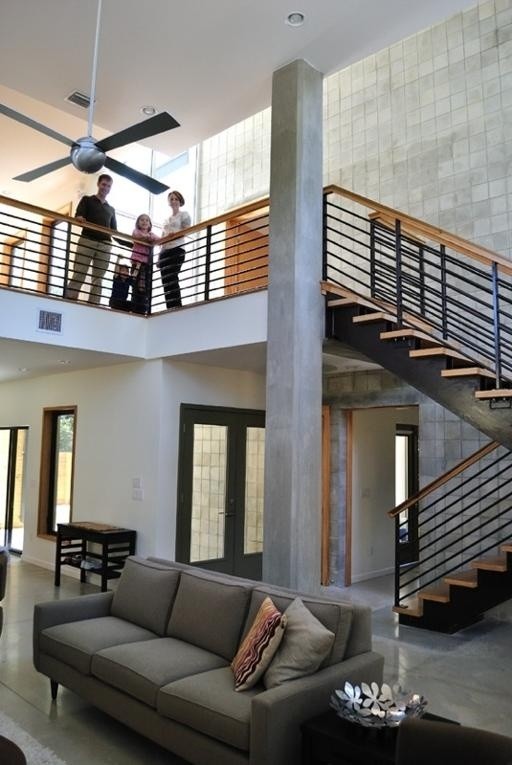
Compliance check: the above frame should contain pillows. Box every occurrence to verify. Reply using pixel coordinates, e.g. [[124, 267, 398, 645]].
[[109, 555, 352, 693]]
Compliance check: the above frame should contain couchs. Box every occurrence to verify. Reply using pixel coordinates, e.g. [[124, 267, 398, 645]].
[[33, 555, 384, 765]]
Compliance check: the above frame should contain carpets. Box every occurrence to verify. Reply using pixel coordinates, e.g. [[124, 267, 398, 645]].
[[0, 710, 68, 765]]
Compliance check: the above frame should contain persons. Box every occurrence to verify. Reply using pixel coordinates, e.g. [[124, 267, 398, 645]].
[[110, 254, 142, 312], [158, 190, 194, 309], [129, 213, 163, 315], [64, 173, 117, 306]]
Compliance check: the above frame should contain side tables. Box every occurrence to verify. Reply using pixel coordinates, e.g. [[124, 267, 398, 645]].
[[300, 693, 461, 765]]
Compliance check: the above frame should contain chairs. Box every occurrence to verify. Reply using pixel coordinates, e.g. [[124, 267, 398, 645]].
[[394, 718, 512, 765]]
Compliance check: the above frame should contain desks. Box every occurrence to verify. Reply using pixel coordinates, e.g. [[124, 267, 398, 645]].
[[55, 522, 136, 592]]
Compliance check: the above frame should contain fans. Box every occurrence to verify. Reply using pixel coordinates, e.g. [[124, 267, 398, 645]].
[[0, 0, 180, 194]]
